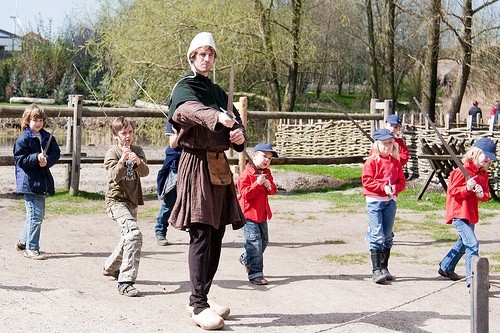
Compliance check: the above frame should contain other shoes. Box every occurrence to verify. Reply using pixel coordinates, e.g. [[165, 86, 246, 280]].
[[191, 308, 225, 330], [249, 276, 268, 285], [187, 299, 230, 318], [381, 269, 393, 281], [372, 270, 387, 283], [438, 268, 462, 281], [156, 234, 168, 246], [27, 249, 46, 260], [469, 284, 491, 294], [15, 242, 29, 258], [239, 254, 250, 273], [118, 283, 141, 297], [102, 266, 120, 280]]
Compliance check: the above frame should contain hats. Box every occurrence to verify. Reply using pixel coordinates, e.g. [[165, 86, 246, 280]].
[[169, 32, 217, 101], [371, 129, 395, 141], [474, 138, 497, 161], [387, 114, 402, 128], [253, 143, 279, 158]]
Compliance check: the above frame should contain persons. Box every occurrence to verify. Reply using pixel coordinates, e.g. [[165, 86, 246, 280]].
[[235, 143, 280, 286], [168, 29, 250, 331], [489, 99, 500, 127], [12, 102, 62, 261], [153, 134, 187, 247], [367, 114, 411, 166], [437, 137, 500, 290], [99, 114, 150, 298], [360, 127, 407, 284], [468, 100, 483, 126]]
